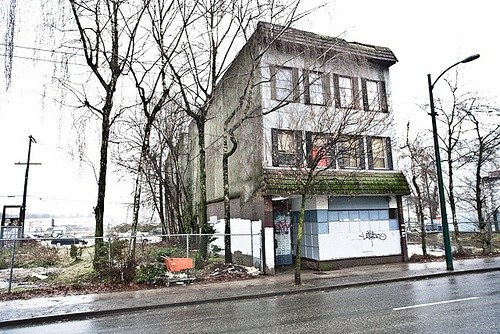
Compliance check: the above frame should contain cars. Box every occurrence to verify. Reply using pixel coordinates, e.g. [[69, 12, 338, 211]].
[[1, 216, 164, 248]]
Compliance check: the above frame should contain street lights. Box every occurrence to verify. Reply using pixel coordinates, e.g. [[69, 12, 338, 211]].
[[424, 51, 481, 271]]
